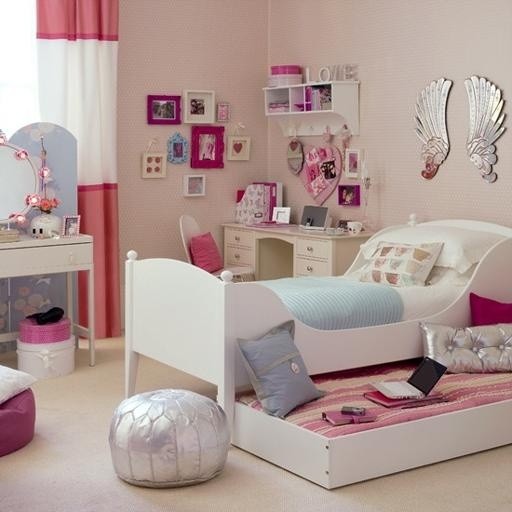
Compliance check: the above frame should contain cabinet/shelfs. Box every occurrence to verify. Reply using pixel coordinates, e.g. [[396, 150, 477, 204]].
[[261, 79, 364, 136]]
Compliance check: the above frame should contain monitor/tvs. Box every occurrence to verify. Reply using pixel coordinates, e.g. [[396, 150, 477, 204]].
[[300, 205, 329, 230]]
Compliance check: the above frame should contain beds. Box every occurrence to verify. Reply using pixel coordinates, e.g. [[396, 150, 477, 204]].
[[122, 213, 512, 491]]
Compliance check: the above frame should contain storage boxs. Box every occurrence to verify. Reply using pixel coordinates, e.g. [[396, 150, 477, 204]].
[[15, 336, 77, 381]]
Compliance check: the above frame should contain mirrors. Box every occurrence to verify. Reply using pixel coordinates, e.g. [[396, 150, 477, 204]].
[[285, 137, 304, 176], [0, 129, 42, 229]]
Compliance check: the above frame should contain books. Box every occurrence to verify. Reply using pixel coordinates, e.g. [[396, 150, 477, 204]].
[[321, 411, 376, 426], [363, 391, 447, 410]]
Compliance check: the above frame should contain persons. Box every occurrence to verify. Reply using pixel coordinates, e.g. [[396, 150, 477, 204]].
[[159, 106, 167, 118], [197, 105, 204, 114], [321, 163, 336, 178], [190, 100, 198, 114], [342, 189, 347, 202], [201, 142, 215, 160]]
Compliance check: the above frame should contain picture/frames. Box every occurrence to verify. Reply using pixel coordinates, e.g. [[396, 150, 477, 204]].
[[166, 132, 189, 165], [227, 136, 250, 161], [218, 102, 231, 123], [271, 207, 291, 224], [183, 174, 207, 198], [190, 125, 226, 169], [145, 93, 182, 124], [184, 91, 216, 124], [337, 184, 361, 206], [63, 215, 81, 237], [343, 148, 362, 180], [142, 154, 166, 179]]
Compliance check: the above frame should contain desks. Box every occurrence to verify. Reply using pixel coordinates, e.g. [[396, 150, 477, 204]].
[[1, 233, 96, 369], [222, 222, 370, 281]]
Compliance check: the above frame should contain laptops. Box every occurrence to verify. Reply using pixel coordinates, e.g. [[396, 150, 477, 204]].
[[367, 355, 447, 400]]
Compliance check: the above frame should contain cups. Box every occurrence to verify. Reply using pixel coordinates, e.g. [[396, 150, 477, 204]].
[[347, 221, 363, 237]]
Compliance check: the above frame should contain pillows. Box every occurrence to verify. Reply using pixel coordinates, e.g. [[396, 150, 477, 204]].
[[419, 322, 512, 373], [189, 231, 226, 275], [238, 321, 327, 420], [359, 241, 443, 286], [470, 293, 512, 325], [360, 224, 504, 274], [0, 363, 37, 406]]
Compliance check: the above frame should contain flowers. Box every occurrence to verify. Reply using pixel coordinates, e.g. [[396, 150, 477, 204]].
[[36, 198, 60, 214]]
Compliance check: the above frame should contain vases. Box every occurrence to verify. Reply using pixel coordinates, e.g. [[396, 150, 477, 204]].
[[32, 215, 62, 236]]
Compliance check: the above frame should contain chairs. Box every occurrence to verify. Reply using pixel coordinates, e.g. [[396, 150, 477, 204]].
[[177, 213, 253, 282]]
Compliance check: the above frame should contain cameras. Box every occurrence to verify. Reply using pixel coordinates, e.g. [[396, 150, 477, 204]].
[[341, 405, 366, 416]]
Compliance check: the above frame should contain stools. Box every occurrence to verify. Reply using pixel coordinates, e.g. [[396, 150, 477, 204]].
[[1, 387, 35, 458], [111, 387, 234, 491]]
